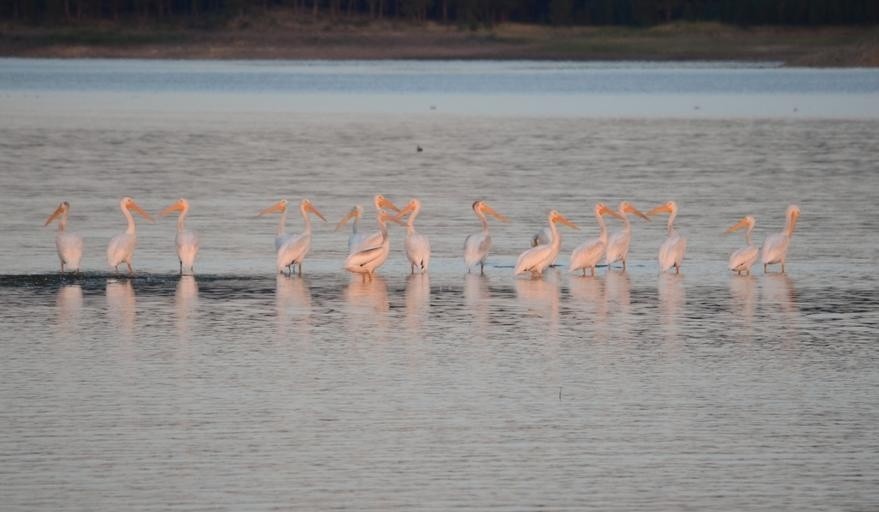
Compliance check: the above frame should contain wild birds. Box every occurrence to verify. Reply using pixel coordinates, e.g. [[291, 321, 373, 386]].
[[106, 195, 153, 273], [252, 196, 328, 279], [514, 199, 688, 281], [331, 194, 432, 285], [719, 202, 802, 277], [461, 200, 503, 275], [157, 197, 200, 278], [43, 201, 84, 277]]
